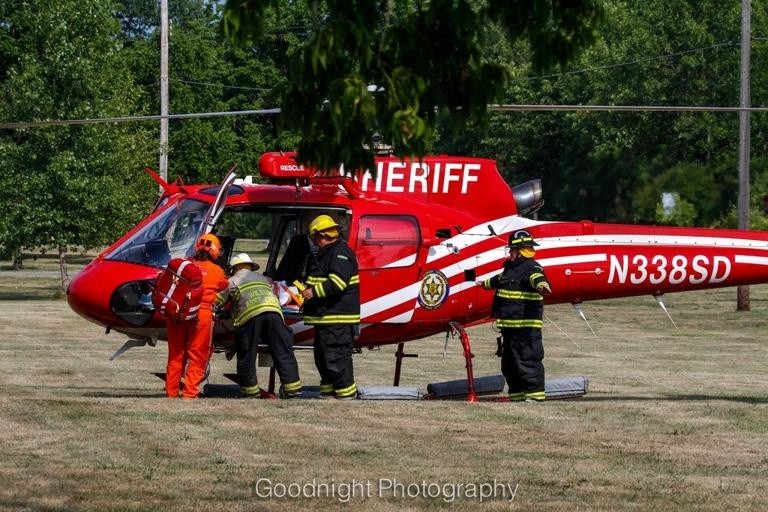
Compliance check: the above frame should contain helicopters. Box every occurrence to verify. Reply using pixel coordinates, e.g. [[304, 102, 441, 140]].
[[0, 85, 768, 401]]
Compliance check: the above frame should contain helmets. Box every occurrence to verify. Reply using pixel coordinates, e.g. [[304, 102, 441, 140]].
[[228, 252, 260, 274], [308, 214, 339, 236], [193, 233, 222, 263], [505, 229, 540, 248]]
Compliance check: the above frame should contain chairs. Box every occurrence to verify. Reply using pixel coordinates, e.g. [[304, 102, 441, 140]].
[[274, 234, 313, 287]]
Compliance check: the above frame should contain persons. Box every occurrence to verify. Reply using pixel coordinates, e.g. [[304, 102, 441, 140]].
[[475, 230, 552, 403], [166, 234, 229, 399], [214, 253, 302, 399], [302, 215, 361, 401]]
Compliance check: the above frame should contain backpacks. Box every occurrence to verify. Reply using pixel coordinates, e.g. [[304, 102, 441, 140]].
[[151, 256, 203, 324]]
